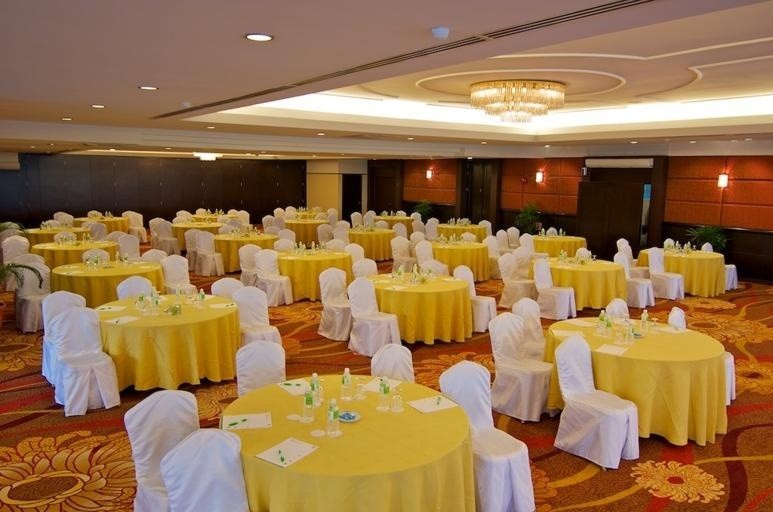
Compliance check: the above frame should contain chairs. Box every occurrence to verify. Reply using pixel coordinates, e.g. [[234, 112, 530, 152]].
[[160, 429, 249, 512], [438, 360, 536, 511], [0, 206, 739, 408], [121, 390, 200, 512]]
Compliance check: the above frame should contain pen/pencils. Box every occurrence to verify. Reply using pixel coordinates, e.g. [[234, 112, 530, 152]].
[[225, 303, 234, 307], [278, 449, 285, 463], [116, 318, 120, 323], [437, 397, 441, 405], [283, 383, 302, 386], [99, 307, 111, 310], [229, 418, 248, 426]]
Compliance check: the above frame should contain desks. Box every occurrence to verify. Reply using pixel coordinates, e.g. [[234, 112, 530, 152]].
[[217, 374, 475, 511]]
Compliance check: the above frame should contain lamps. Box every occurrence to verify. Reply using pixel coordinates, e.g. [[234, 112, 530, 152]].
[[717, 174, 728, 189], [425, 167, 432, 182], [468, 80, 568, 126], [535, 170, 544, 185]]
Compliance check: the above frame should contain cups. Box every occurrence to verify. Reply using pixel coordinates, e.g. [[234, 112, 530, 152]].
[[352, 383, 368, 401]]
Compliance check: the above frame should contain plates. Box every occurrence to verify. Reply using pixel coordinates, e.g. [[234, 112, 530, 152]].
[[287, 413, 300, 421], [310, 428, 324, 438], [338, 412, 361, 423]]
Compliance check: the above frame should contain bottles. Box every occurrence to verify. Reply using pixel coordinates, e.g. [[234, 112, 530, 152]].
[[391, 262, 434, 285], [340, 367, 351, 401], [83, 251, 130, 273], [53, 232, 92, 248], [325, 398, 340, 437], [135, 283, 206, 317], [375, 374, 405, 413], [286, 240, 327, 257], [309, 372, 321, 407], [301, 386, 314, 423], [598, 309, 649, 345]]
